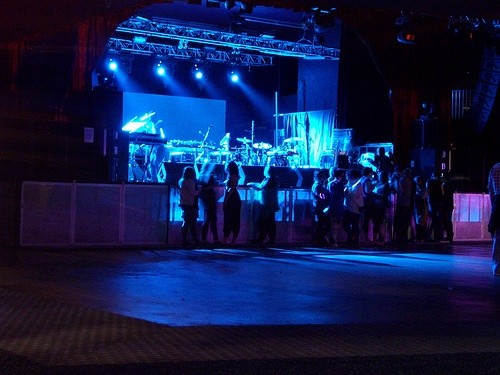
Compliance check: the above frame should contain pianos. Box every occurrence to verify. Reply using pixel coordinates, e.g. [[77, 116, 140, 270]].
[[129, 132, 167, 145]]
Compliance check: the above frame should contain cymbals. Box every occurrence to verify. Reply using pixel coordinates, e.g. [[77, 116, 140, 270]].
[[284, 137, 302, 142], [236, 138, 252, 143], [253, 142, 272, 149]]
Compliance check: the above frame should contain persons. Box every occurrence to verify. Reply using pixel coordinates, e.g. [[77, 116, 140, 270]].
[[218, 160, 242, 246], [363, 147, 454, 244], [311, 171, 333, 245], [343, 171, 363, 247], [330, 168, 348, 245], [247, 166, 278, 248], [197, 163, 223, 249], [177, 166, 199, 250], [487, 162, 499, 275]]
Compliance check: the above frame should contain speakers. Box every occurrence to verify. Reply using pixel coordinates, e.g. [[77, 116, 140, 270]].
[[157, 162, 319, 189]]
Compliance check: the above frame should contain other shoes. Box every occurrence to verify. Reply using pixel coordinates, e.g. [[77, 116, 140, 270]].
[[318, 236, 455, 252], [493, 272, 500, 275], [180, 239, 276, 249]]
